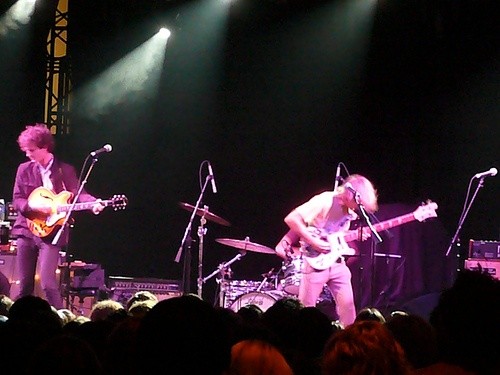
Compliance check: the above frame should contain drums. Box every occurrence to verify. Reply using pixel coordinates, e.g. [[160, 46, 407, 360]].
[[281, 255, 326, 298], [218, 277, 274, 310], [226, 289, 292, 313]]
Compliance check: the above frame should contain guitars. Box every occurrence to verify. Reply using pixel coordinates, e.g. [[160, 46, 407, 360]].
[[300, 199, 441, 272], [25, 185, 129, 239]]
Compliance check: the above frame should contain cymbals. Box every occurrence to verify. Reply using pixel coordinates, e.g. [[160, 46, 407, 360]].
[[340, 250, 366, 259], [214, 236, 276, 255], [176, 200, 231, 229]]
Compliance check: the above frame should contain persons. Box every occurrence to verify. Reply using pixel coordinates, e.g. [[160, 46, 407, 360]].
[[276, 229, 302, 290], [12, 123, 104, 309], [0, 263, 500, 375], [284, 175, 379, 328]]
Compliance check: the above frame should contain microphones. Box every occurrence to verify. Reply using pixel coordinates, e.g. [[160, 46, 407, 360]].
[[334, 167, 340, 193], [475, 168, 498, 178], [208, 165, 217, 193], [90, 144, 112, 156]]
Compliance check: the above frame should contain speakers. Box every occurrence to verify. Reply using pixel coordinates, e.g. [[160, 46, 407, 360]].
[[0, 250, 67, 301], [105, 277, 182, 305]]
